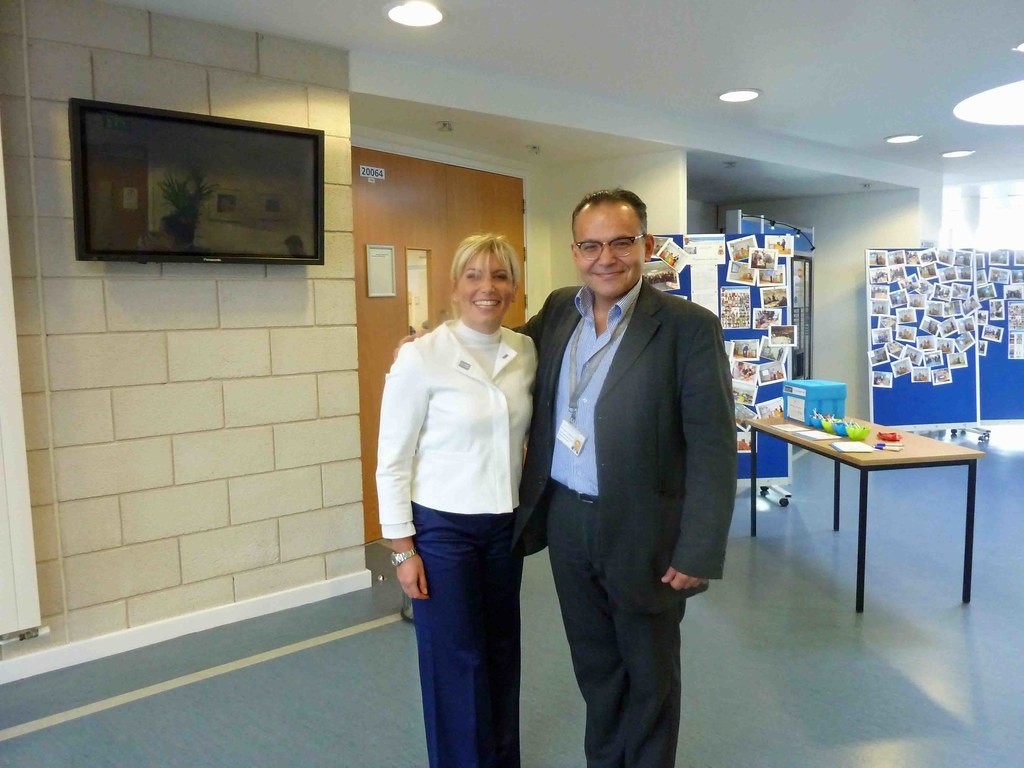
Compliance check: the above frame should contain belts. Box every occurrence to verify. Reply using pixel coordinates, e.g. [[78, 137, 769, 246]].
[[550, 479, 599, 505]]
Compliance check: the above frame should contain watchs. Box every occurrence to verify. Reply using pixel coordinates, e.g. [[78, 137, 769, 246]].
[[391, 549, 417, 566]]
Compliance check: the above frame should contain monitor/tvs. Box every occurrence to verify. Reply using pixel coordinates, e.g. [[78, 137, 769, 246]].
[[67, 95, 327, 265]]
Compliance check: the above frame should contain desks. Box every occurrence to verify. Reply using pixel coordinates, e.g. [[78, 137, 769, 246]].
[[746, 417, 986, 613]]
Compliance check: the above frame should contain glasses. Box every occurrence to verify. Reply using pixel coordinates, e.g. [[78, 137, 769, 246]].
[[575, 233, 645, 261]]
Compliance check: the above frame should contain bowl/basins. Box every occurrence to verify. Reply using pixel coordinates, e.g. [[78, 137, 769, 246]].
[[821, 419, 844, 433], [832, 422, 856, 436], [846, 425, 871, 441], [810, 417, 824, 429]]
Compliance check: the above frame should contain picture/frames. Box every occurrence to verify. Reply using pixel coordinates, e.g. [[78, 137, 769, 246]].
[[210, 188, 247, 222]]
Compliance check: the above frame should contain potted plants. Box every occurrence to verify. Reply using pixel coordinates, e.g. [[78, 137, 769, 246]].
[[155, 164, 219, 243]]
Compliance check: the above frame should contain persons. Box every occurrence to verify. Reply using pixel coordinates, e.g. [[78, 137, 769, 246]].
[[721, 238, 791, 451], [694, 247, 697, 254], [643, 272, 675, 284], [375, 235, 537, 768], [394, 191, 738, 768], [870, 252, 1024, 386], [571, 441, 580, 454], [655, 245, 679, 266]]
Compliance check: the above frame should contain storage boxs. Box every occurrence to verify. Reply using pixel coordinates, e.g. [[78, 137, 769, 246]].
[[782, 379, 847, 426]]
